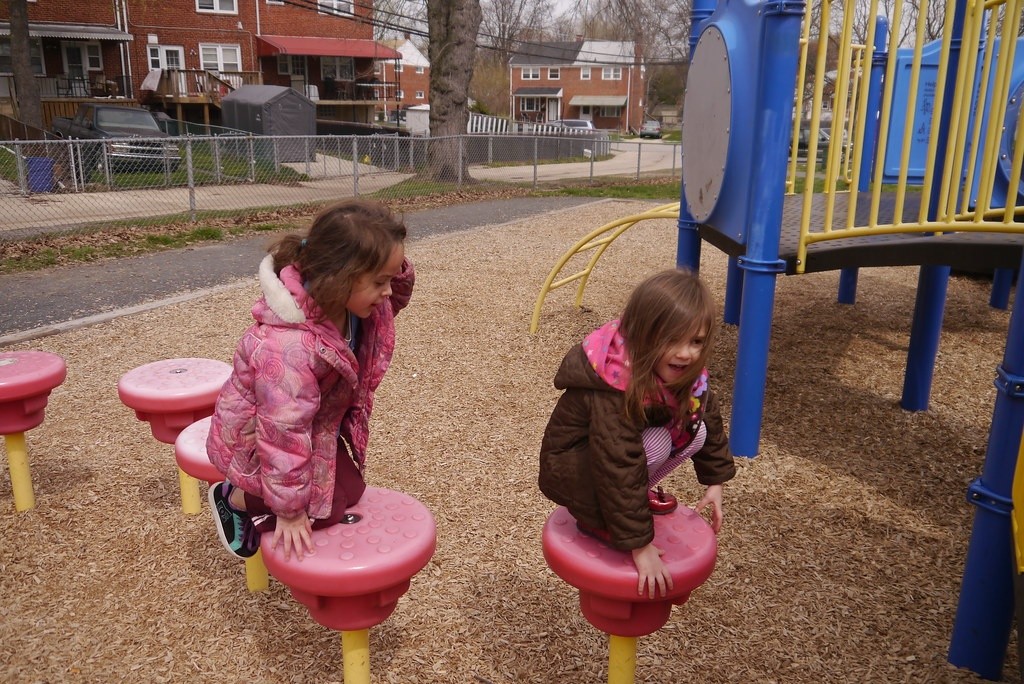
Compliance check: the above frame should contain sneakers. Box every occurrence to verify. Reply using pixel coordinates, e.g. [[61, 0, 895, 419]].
[[207, 481, 261, 560]]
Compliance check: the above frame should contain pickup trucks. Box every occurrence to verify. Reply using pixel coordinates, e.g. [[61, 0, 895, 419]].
[[49, 102, 182, 174]]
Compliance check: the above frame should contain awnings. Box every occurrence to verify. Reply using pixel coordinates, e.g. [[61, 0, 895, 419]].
[[513, 87, 563, 97], [28, 24, 133, 43], [569, 95, 627, 106], [257, 35, 403, 63]]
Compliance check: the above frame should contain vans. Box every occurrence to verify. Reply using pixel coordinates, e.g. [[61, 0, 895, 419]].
[[552, 119, 597, 132]]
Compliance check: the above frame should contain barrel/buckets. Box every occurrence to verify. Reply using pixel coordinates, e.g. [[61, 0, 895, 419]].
[[25, 157, 55, 194]]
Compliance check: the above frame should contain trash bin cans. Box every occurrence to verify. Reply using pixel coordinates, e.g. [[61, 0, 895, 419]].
[[27, 157, 55, 192]]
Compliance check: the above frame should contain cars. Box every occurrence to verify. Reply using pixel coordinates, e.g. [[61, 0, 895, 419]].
[[639, 121, 662, 139], [789, 128, 853, 164]]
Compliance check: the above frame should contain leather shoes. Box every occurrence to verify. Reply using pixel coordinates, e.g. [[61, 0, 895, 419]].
[[578, 518, 615, 546], [648, 485, 678, 514]]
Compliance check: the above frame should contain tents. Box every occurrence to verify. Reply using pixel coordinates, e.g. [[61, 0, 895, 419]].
[[221, 85, 317, 162]]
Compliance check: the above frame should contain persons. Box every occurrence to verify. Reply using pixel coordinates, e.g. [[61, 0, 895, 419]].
[[538, 272, 735, 598], [206, 198, 415, 558]]
